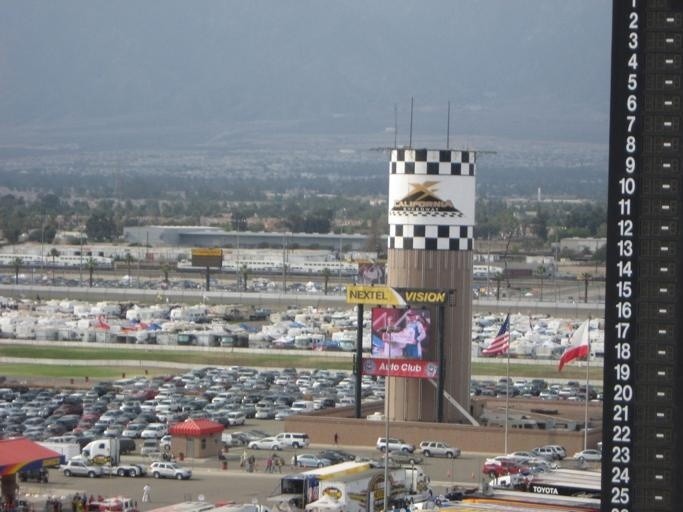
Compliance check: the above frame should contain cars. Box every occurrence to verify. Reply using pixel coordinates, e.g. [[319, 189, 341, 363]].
[[222, 431, 311, 451], [573, 449, 602, 463], [194, 366, 385, 426], [481, 445, 567, 479], [469, 377, 604, 403], [146, 500, 271, 512], [291, 450, 355, 468], [0, 372, 199, 454]]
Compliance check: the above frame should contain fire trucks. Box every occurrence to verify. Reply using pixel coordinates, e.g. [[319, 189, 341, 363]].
[[88, 496, 138, 512]]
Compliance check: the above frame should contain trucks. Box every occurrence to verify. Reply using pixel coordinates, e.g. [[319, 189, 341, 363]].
[[281, 459, 431, 512]]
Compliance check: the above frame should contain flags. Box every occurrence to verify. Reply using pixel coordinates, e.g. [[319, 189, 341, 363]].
[[479, 313, 508, 357], [557, 318, 589, 371]]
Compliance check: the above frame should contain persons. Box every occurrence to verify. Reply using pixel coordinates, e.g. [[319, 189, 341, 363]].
[[0, 487, 104, 511], [371, 308, 429, 358], [215, 444, 299, 475], [332, 432, 339, 444], [141, 482, 151, 503]]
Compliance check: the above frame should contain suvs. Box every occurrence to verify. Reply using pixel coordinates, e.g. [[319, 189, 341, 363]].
[[376, 437, 415, 454], [419, 441, 460, 459], [62, 455, 192, 480]]
